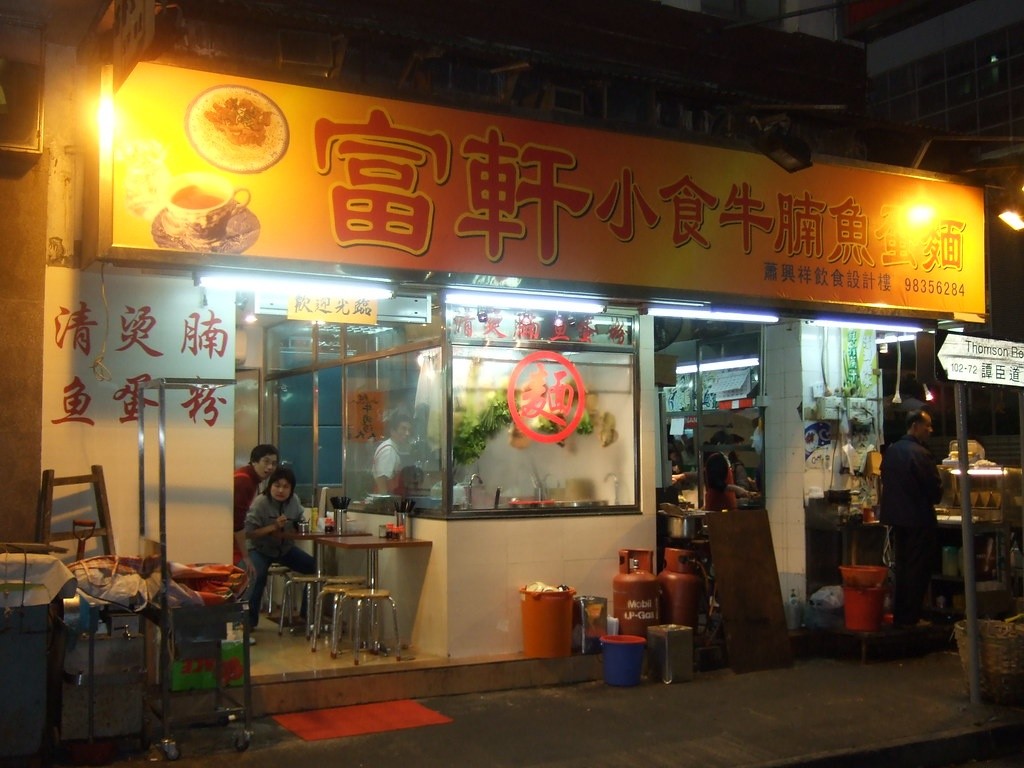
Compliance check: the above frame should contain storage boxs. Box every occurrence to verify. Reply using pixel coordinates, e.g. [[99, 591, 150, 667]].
[[952, 582, 1011, 617], [573, 595, 607, 655], [167, 638, 255, 690], [647, 624, 693, 685]]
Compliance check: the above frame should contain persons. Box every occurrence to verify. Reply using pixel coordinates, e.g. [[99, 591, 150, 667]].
[[233, 444, 280, 630], [371, 414, 413, 515], [239, 467, 316, 633], [657, 422, 760, 607], [878, 410, 946, 628]]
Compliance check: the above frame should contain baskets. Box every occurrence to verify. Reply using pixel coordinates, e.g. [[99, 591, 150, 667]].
[[955, 614, 1024, 705]]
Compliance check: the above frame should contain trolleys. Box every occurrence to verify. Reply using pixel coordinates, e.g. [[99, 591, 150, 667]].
[[137, 378, 255, 761]]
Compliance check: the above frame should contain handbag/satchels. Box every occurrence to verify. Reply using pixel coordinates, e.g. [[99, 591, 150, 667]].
[[0, 553, 250, 614]]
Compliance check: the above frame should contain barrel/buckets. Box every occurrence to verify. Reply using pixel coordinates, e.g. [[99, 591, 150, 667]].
[[519, 585, 576, 658], [839, 565, 887, 631], [600, 636, 646, 687]]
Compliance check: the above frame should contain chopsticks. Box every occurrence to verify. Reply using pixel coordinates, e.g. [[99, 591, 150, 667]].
[[330, 496, 350, 509], [268, 516, 303, 523], [394, 499, 415, 513]]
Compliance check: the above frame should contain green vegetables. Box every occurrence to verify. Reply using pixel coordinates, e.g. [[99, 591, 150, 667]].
[[454, 379, 595, 465]]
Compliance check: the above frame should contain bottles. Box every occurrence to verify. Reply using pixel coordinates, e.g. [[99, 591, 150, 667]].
[[379, 525, 386, 537], [325, 519, 333, 533], [392, 527, 399, 540], [311, 508, 318, 532], [386, 523, 393, 539]]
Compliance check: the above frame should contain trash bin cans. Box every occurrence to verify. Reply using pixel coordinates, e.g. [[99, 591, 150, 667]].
[[599, 635, 647, 687], [573, 596, 607, 654], [648, 624, 694, 684]]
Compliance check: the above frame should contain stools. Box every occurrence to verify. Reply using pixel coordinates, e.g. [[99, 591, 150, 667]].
[[265, 564, 401, 665]]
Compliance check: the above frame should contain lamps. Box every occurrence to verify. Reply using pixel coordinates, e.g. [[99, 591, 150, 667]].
[[923, 384, 933, 400], [637, 303, 778, 323], [805, 319, 936, 335], [191, 272, 396, 300], [445, 291, 608, 315], [750, 113, 814, 173], [893, 342, 902, 403]]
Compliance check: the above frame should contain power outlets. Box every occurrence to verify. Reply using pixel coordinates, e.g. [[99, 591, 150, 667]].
[[846, 398, 867, 421], [816, 395, 842, 420]]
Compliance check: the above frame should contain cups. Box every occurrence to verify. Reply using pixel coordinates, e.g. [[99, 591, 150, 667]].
[[165, 183, 251, 245], [333, 509, 347, 535], [396, 513, 411, 538]]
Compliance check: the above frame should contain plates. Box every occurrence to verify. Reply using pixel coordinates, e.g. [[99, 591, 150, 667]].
[[184, 84, 290, 173], [151, 199, 259, 255]]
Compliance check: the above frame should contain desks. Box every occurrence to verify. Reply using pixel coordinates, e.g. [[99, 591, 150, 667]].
[[848, 519, 1012, 595], [270, 529, 432, 657]]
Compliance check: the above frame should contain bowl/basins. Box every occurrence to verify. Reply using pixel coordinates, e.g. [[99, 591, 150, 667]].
[[299, 524, 309, 532]]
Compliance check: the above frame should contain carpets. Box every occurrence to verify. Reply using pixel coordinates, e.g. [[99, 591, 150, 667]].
[[270, 699, 453, 742]]
